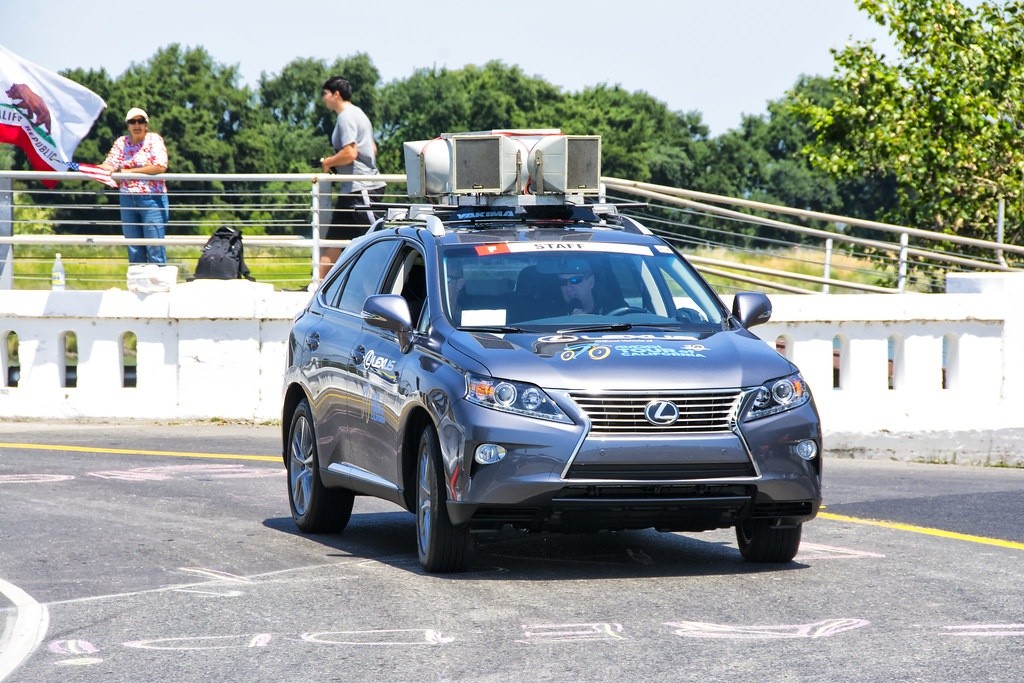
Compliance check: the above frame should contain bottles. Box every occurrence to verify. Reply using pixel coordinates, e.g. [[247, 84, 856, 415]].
[[53, 253, 65, 290]]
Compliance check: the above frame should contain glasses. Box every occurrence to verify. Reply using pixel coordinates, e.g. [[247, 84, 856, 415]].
[[127, 119, 145, 124], [446, 276, 461, 282], [556, 275, 594, 284]]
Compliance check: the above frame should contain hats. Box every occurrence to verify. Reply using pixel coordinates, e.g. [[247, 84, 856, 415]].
[[125, 108, 149, 123]]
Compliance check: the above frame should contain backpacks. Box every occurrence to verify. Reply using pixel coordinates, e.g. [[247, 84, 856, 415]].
[[194, 226, 256, 282]]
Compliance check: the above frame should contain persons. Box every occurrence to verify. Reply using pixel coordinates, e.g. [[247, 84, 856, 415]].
[[549, 259, 629, 317], [425, 261, 461, 333], [96, 108, 170, 267], [307, 76, 386, 292]]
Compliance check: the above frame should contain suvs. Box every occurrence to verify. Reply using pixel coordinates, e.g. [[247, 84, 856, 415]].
[[280, 129, 823, 574]]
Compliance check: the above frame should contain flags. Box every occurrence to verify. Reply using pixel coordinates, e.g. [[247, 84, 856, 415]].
[[0, 45, 105, 189], [56, 159, 117, 186]]
